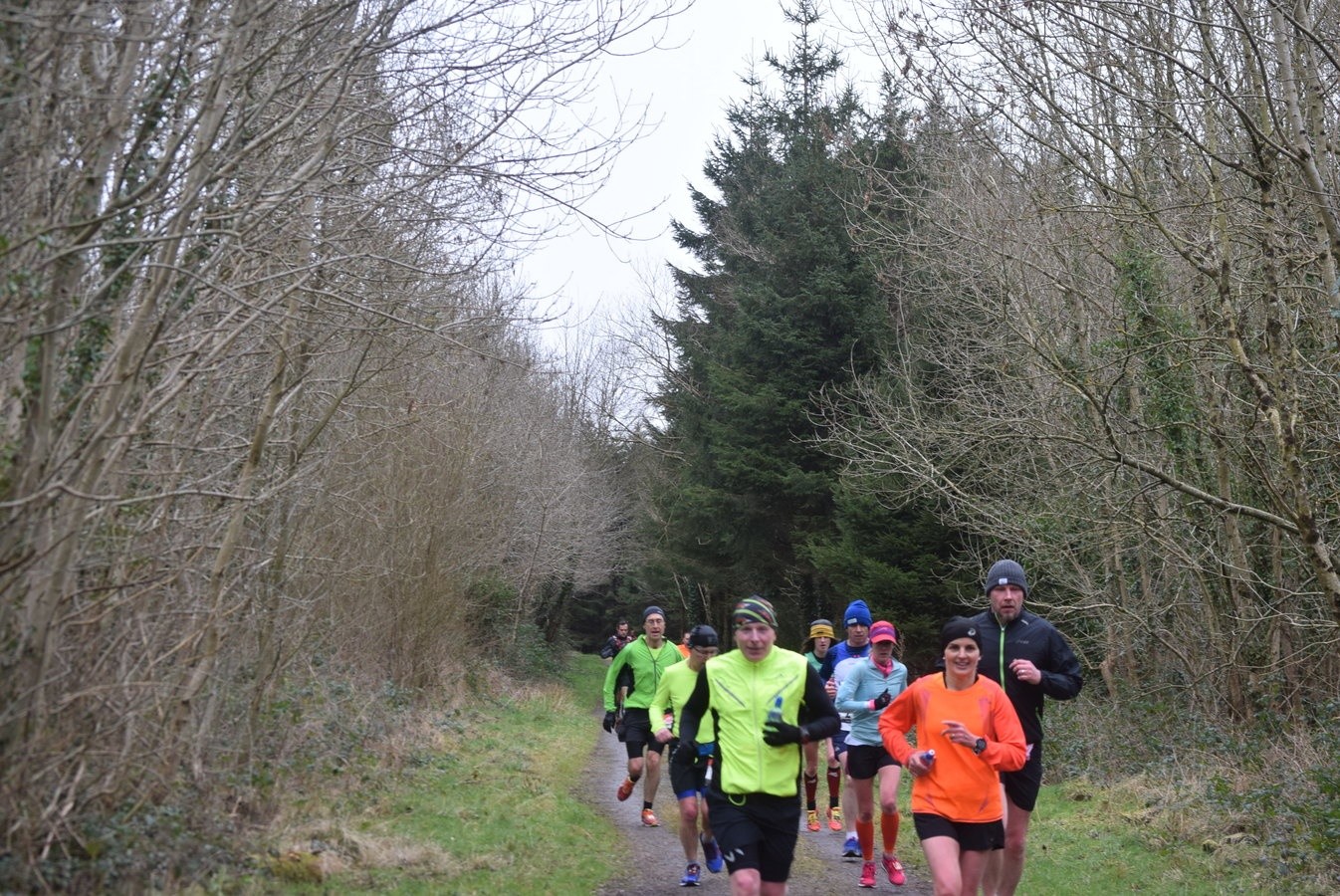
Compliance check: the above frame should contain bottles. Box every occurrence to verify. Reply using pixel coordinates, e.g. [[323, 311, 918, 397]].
[[911, 749, 936, 777], [767, 697, 783, 723], [829, 672, 836, 700]]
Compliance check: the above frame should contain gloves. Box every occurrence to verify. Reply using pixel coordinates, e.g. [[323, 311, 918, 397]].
[[603, 711, 615, 733], [762, 718, 802, 745], [682, 740, 697, 763], [874, 688, 890, 710]]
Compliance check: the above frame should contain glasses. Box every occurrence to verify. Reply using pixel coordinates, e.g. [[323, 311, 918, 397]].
[[692, 646, 720, 656]]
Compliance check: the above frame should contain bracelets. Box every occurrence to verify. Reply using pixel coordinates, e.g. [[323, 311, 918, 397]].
[[869, 699, 875, 711]]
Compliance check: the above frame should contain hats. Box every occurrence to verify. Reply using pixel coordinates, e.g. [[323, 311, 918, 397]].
[[809, 619, 841, 644], [643, 606, 664, 620], [732, 596, 778, 632], [843, 601, 874, 628], [868, 619, 899, 647], [690, 625, 718, 647], [942, 617, 982, 653], [985, 559, 1027, 598]]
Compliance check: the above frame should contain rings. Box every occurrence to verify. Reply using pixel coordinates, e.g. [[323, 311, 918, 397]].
[[1022, 669, 1025, 674]]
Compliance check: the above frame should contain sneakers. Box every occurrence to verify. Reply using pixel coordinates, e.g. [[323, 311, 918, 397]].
[[859, 859, 877, 888], [681, 861, 701, 887], [641, 809, 660, 827], [826, 806, 842, 831], [880, 854, 905, 885], [699, 832, 723, 873], [618, 775, 639, 802], [842, 839, 860, 856]]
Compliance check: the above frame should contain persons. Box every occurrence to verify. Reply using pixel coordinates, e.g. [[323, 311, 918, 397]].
[[600, 619, 635, 663], [835, 621, 908, 888], [602, 606, 686, 827], [801, 619, 844, 832], [877, 616, 1026, 896], [648, 625, 724, 887], [936, 560, 1083, 896], [825, 600, 873, 863], [670, 596, 841, 896], [675, 633, 692, 658]]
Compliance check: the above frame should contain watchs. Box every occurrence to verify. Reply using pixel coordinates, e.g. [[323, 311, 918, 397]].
[[802, 725, 811, 743], [973, 737, 986, 754]]
